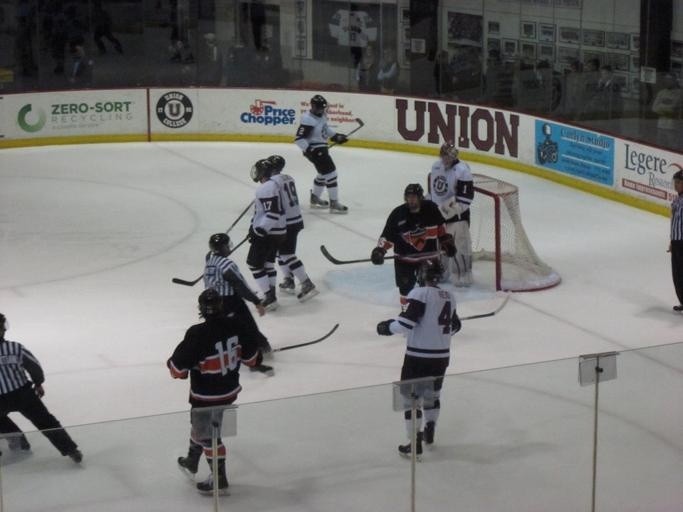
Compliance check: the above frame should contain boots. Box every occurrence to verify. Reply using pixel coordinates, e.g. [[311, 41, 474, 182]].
[[309, 194, 330, 209], [7, 437, 33, 455], [260, 295, 280, 314], [278, 277, 296, 295], [673, 305, 683, 313], [196, 472, 230, 497], [70, 450, 83, 468], [296, 279, 319, 302], [420, 427, 434, 444], [329, 201, 348, 215], [178, 456, 199, 481], [399, 437, 423, 462]]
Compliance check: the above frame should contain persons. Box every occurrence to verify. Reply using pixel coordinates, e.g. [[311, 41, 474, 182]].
[[370, 183, 458, 310], [203, 233, 274, 378], [354, 44, 379, 92], [63, 32, 278, 91], [377, 259, 461, 458], [428, 44, 624, 121], [666, 170, 683, 312], [245, 159, 287, 312], [376, 47, 400, 96], [0, 315, 86, 463], [651, 72, 683, 152], [424, 143, 474, 286], [293, 94, 349, 213], [167, 287, 263, 495], [266, 155, 320, 303]]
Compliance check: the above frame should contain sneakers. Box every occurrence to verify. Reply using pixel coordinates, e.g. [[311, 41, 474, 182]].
[[249, 364, 274, 379]]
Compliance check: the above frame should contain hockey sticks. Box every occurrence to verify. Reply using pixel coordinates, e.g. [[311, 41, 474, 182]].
[[273, 323, 339, 353], [459, 294, 513, 322], [328, 117, 364, 149], [172, 234, 250, 287], [320, 245, 446, 265], [205, 201, 255, 265]]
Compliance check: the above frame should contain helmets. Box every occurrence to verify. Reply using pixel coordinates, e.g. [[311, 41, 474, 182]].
[[0, 313, 8, 338], [310, 94, 328, 116], [198, 289, 224, 318], [208, 233, 230, 254], [249, 159, 273, 183], [268, 155, 286, 172], [439, 141, 459, 162], [404, 183, 426, 202], [414, 258, 445, 284]]
